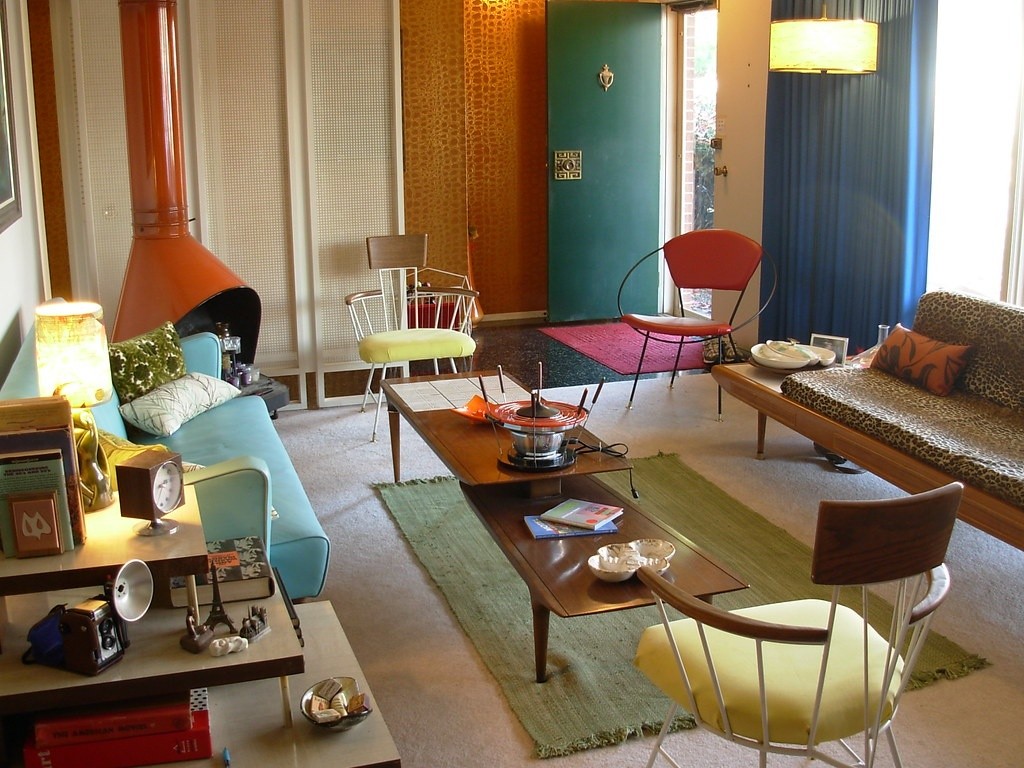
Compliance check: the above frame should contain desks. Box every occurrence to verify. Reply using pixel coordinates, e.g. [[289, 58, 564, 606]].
[[379, 370, 752, 684], [238, 372, 290, 420]]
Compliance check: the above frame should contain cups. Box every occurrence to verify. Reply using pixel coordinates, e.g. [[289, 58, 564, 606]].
[[225, 360, 260, 391]]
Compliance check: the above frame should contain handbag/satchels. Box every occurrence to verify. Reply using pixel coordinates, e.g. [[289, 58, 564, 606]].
[[73, 409, 114, 512]]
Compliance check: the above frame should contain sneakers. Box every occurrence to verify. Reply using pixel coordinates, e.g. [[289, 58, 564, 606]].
[[702, 336, 719, 363], [721, 334, 745, 361]]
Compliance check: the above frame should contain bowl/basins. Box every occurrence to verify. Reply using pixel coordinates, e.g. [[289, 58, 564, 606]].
[[300, 677, 373, 733], [588, 537, 675, 581]]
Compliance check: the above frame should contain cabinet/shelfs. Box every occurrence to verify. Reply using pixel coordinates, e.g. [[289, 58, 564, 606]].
[[0, 485, 403, 768]]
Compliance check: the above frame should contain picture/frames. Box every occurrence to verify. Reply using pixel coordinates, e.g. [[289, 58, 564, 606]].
[[810, 332, 850, 367], [0, 0, 23, 235]]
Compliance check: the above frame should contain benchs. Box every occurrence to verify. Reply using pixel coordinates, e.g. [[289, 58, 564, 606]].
[[0, 296, 332, 604], [711, 286, 1024, 553]]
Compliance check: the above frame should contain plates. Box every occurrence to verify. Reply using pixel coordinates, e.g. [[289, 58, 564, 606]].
[[751, 340, 837, 373]]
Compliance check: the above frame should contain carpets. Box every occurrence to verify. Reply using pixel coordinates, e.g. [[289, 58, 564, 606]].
[[371, 449, 994, 761], [537, 321, 706, 376]]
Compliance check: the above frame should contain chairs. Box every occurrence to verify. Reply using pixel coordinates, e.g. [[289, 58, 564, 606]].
[[617, 229, 778, 423], [344, 233, 480, 443], [633, 480, 964, 768]]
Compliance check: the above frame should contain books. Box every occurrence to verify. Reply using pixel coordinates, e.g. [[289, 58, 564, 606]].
[[169, 536, 276, 608], [524, 516, 618, 540], [539, 498, 624, 531], [0, 394, 87, 559], [21, 686, 213, 768]]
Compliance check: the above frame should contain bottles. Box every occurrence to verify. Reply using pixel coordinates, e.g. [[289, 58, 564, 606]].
[[850, 324, 890, 371]]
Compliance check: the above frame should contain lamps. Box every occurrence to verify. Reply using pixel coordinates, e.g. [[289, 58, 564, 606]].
[[32, 301, 116, 515], [767, 2, 881, 335]]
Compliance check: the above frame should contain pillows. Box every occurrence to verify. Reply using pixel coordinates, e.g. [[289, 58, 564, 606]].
[[118, 371, 242, 438], [871, 321, 973, 397], [108, 320, 188, 406], [97, 428, 280, 521]]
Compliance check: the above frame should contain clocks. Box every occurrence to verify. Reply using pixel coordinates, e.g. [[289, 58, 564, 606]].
[[115, 449, 185, 537]]
